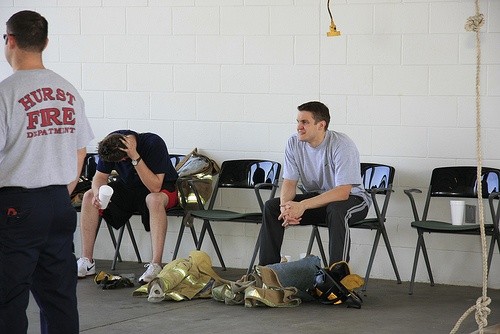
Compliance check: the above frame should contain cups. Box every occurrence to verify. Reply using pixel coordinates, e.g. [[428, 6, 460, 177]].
[[98, 185, 114, 210], [450, 200, 465, 226]]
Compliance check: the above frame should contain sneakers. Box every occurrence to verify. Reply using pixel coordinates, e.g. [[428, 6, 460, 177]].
[[137, 262, 163, 284], [76, 256, 96, 278]]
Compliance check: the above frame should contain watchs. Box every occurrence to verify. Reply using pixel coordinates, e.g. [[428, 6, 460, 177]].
[[132, 157, 141, 165]]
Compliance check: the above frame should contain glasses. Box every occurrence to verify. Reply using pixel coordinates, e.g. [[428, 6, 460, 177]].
[[3, 34, 19, 45]]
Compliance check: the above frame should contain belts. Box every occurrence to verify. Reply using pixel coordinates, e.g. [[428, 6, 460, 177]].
[[0, 184, 67, 193]]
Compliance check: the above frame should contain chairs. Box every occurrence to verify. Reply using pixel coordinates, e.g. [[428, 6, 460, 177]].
[[404, 167, 500, 295], [306, 164, 401, 296], [187, 159, 282, 276], [69, 153, 122, 262], [111, 154, 198, 271]]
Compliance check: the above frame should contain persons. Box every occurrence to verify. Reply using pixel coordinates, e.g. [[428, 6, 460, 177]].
[[77, 130, 179, 283], [259, 101, 373, 268], [0, 10, 94, 334]]
